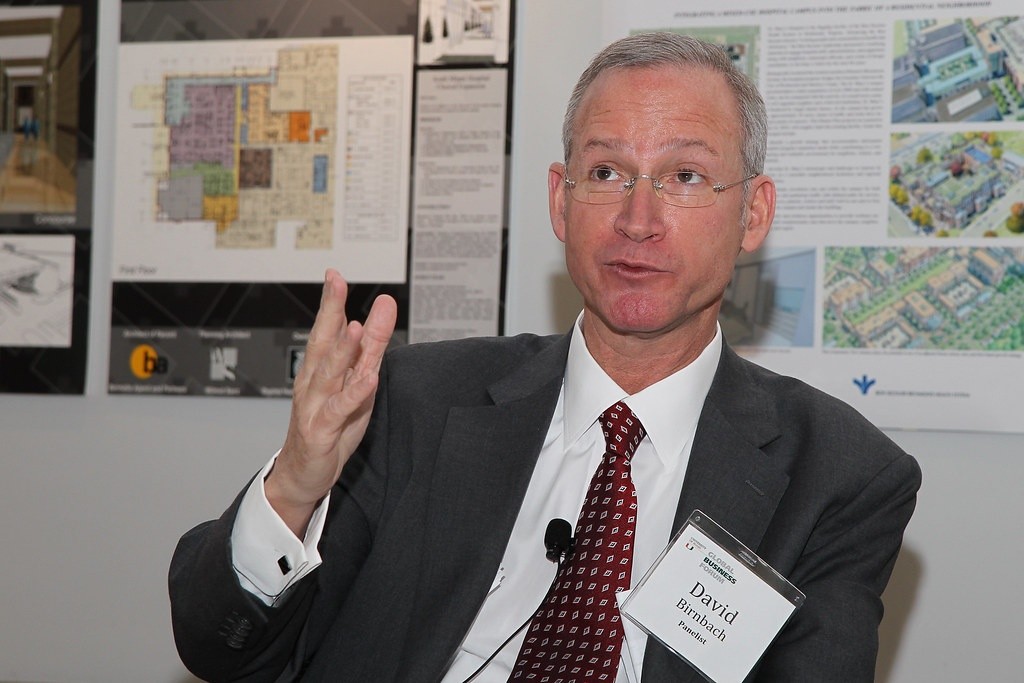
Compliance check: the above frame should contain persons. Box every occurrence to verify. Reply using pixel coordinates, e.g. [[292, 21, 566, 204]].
[[167, 33, 923, 682]]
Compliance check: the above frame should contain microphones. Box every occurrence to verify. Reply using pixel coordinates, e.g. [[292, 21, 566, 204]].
[[544, 518, 572, 564]]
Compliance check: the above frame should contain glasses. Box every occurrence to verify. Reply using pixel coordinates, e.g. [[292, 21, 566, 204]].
[[567, 167, 759, 209]]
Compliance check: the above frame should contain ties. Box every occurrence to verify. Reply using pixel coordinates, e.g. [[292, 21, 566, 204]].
[[507, 401, 645, 682]]
